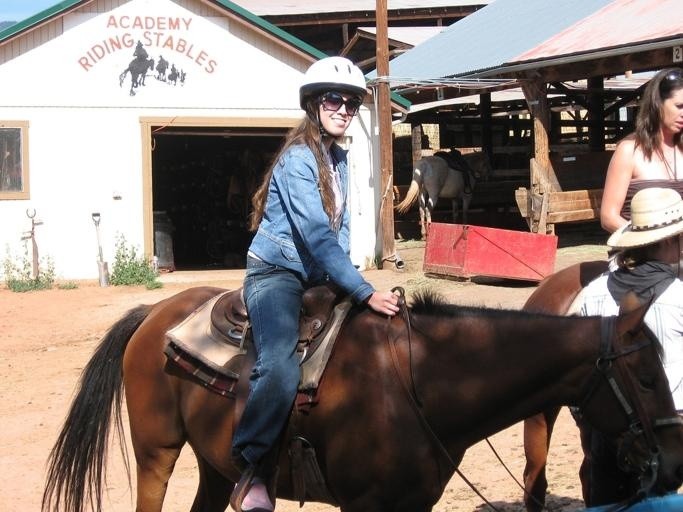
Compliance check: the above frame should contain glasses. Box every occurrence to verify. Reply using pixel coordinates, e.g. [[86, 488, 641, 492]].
[[309, 90, 362, 117], [660, 71, 683, 87]]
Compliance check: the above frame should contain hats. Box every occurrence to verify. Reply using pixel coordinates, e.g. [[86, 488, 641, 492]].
[[605, 187, 683, 249]]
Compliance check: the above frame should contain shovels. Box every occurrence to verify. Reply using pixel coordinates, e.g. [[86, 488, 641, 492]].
[[92, 212, 110, 288]]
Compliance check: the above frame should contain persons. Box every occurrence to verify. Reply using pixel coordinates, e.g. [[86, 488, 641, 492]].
[[180, 68, 183, 73], [169, 63, 176, 73], [158, 55, 164, 62], [571, 187, 683, 418], [132, 39, 147, 60], [598, 65, 683, 271], [230, 55, 398, 512]]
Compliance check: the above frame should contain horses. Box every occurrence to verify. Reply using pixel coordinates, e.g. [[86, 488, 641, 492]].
[[522, 261, 683, 511], [393, 147, 492, 241], [39, 285, 683, 512]]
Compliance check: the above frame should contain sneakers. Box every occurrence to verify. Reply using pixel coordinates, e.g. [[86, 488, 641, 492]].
[[231, 473, 276, 511]]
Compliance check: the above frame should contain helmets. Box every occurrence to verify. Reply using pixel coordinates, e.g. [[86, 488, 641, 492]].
[[299, 55, 368, 107]]
